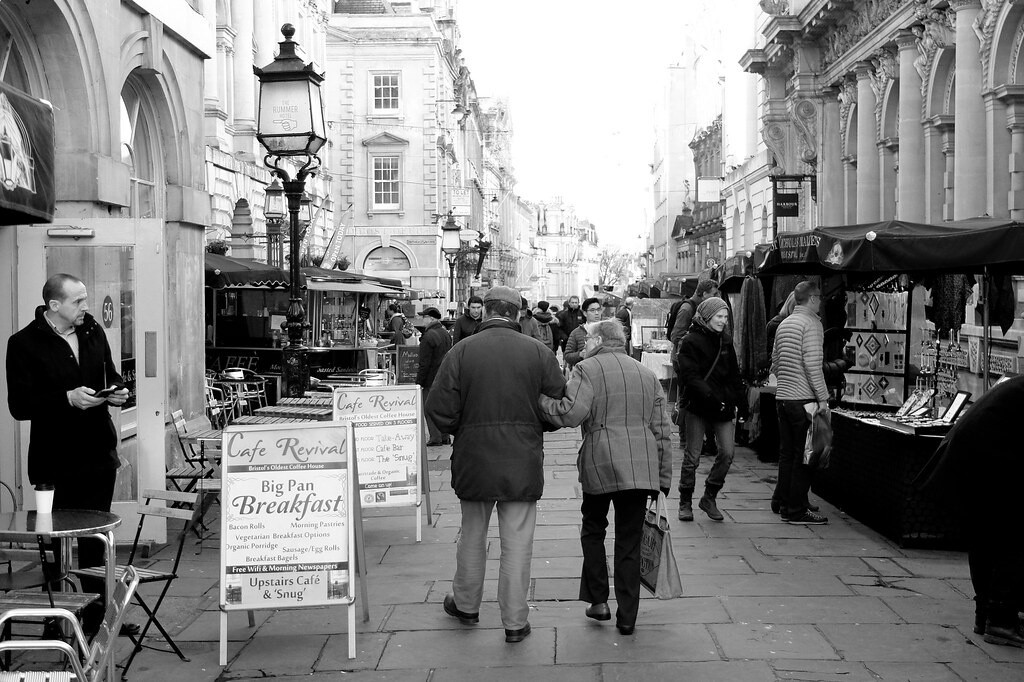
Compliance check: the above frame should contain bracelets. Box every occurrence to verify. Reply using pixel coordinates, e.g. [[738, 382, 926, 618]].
[[67, 390, 74, 407]]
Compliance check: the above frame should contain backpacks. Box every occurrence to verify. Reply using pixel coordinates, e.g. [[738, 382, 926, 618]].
[[400, 314, 414, 339], [665, 299, 697, 342]]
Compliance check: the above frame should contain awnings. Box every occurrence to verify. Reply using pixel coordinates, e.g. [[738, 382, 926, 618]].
[[297, 264, 426, 300]]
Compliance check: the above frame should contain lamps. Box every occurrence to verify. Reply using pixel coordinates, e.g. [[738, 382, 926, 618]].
[[436, 99, 464, 120]]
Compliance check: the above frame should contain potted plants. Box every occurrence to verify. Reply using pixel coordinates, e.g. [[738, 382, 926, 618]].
[[206, 241, 228, 255], [338, 256, 350, 270]]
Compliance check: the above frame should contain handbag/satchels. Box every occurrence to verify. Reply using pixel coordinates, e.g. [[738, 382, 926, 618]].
[[640, 491, 683, 601], [802, 401, 833, 470], [671, 400, 686, 426]]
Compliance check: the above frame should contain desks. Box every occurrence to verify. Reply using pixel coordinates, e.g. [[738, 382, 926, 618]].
[[175, 429, 222, 541], [214, 379, 267, 419], [632, 346, 671, 382], [0, 508, 123, 682], [662, 363, 675, 403], [811, 407, 968, 552], [232, 372, 380, 426]]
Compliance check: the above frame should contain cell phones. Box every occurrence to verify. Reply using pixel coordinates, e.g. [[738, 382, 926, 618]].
[[94, 388, 115, 398]]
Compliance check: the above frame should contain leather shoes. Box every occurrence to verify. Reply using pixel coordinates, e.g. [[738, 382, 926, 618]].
[[505, 620, 531, 642], [616, 620, 634, 634], [984, 618, 1024, 648], [443, 593, 479, 624], [442, 438, 450, 444], [427, 440, 442, 446], [586, 603, 611, 621], [974, 614, 986, 634]]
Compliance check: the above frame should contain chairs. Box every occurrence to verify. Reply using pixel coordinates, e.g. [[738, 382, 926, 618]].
[[0, 481, 200, 682], [164, 365, 269, 540]]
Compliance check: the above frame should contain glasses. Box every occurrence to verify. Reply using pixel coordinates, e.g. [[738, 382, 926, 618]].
[[810, 294, 823, 300], [585, 308, 602, 313], [584, 335, 605, 344]]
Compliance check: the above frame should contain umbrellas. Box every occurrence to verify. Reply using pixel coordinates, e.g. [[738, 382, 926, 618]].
[[204, 252, 290, 347]]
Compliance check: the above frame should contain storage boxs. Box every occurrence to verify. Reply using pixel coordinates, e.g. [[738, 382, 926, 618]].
[[879, 414, 954, 437]]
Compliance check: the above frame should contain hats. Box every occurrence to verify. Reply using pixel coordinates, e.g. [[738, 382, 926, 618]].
[[484, 286, 522, 311], [698, 297, 730, 323], [417, 307, 441, 319]]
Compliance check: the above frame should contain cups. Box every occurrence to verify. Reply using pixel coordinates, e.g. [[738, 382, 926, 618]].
[[34, 483, 55, 513]]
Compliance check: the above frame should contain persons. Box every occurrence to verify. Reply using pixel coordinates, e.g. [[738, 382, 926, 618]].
[[424, 286, 568, 640], [361, 296, 642, 446], [672, 278, 854, 513], [674, 297, 740, 521], [773, 281, 831, 524], [918, 374, 1024, 648], [6, 273, 142, 635], [540, 320, 673, 636]]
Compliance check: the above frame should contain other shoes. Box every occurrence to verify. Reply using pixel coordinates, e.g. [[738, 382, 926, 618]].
[[43, 622, 62, 639], [704, 444, 718, 456], [82, 622, 140, 637], [680, 436, 687, 446]]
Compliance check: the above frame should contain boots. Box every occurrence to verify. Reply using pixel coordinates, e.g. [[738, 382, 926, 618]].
[[678, 488, 693, 520], [698, 485, 724, 520]]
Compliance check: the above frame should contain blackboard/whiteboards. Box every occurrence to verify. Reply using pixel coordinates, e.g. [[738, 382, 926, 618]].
[[396, 345, 420, 384]]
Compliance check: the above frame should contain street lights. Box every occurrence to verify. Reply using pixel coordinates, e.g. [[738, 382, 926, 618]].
[[254, 23, 327, 397], [441, 211, 461, 328]]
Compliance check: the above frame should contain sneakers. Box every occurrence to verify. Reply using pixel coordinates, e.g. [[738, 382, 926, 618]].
[[808, 502, 820, 509], [781, 508, 828, 525]]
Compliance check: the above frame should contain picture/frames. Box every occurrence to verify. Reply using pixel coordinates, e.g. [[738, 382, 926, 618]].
[[910, 406, 932, 415], [941, 390, 972, 422]]
